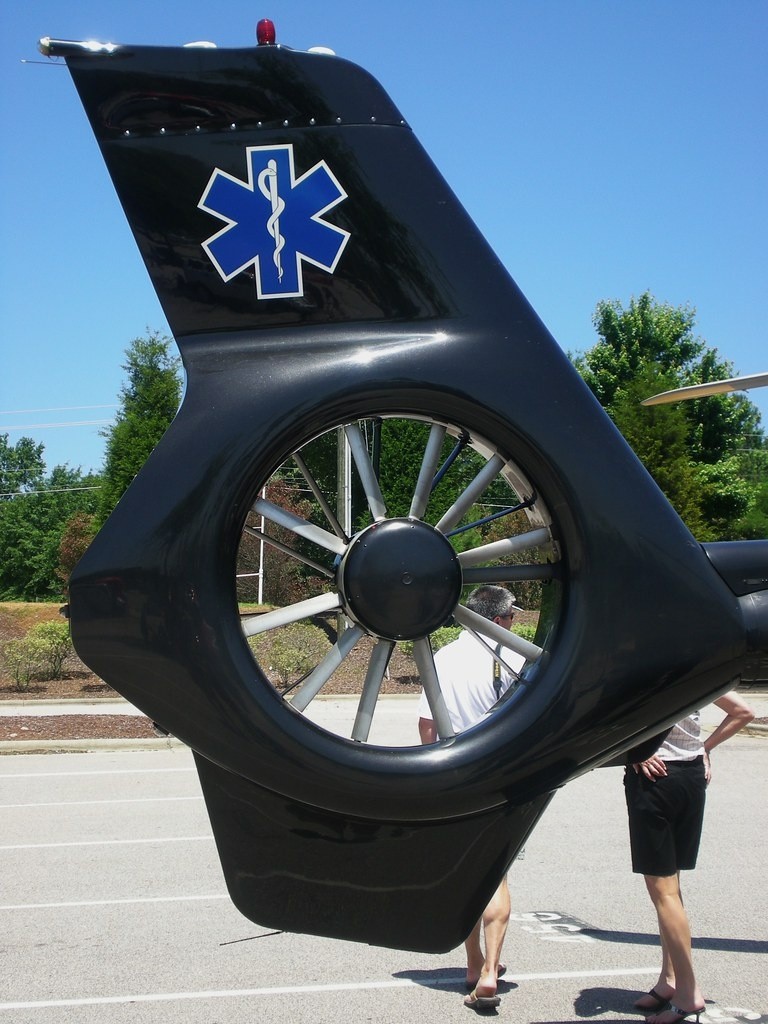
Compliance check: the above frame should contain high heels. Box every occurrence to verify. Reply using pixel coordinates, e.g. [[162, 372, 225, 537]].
[[637, 999, 707, 1023], [631, 988, 672, 1011]]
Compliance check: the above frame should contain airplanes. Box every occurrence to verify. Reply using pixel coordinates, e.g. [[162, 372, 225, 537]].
[[20, 14, 768, 958]]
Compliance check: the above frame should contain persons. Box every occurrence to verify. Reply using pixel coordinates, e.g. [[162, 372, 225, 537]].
[[623, 690, 755, 1024], [419, 586, 531, 1006]]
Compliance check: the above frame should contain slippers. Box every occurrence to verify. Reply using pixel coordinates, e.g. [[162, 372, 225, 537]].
[[464, 989, 504, 1016], [465, 962, 507, 992]]
